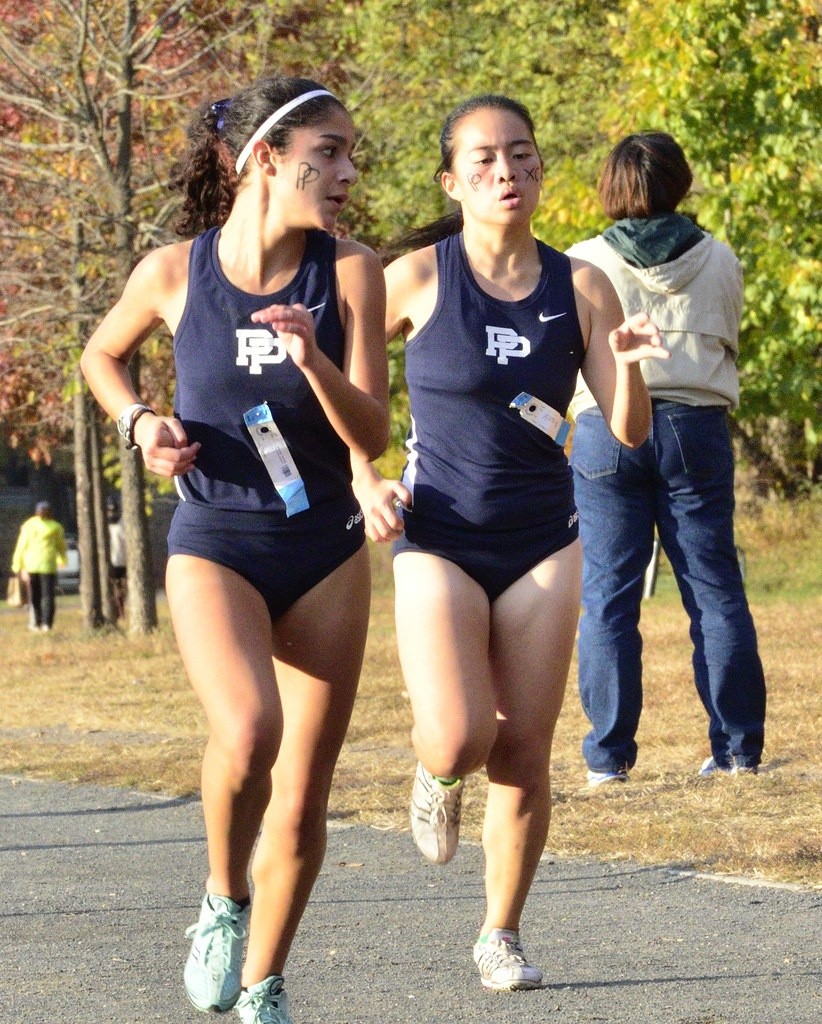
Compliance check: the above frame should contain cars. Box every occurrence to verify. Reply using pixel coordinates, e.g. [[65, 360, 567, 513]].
[[52, 549, 82, 594]]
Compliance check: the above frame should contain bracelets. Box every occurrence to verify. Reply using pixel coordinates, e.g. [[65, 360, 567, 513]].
[[111, 403, 153, 448]]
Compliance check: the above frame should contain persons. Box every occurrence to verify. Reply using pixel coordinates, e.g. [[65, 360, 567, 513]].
[[101, 501, 130, 617], [352, 95, 674, 995], [76, 75, 392, 1020], [560, 127, 765, 784], [10, 498, 74, 632]]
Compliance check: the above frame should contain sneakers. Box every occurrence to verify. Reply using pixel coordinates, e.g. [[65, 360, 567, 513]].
[[700, 756, 760, 776], [411, 760, 462, 864], [182, 893, 251, 1012], [235, 974, 291, 1024], [584, 769, 628, 787], [472, 931, 543, 991]]
